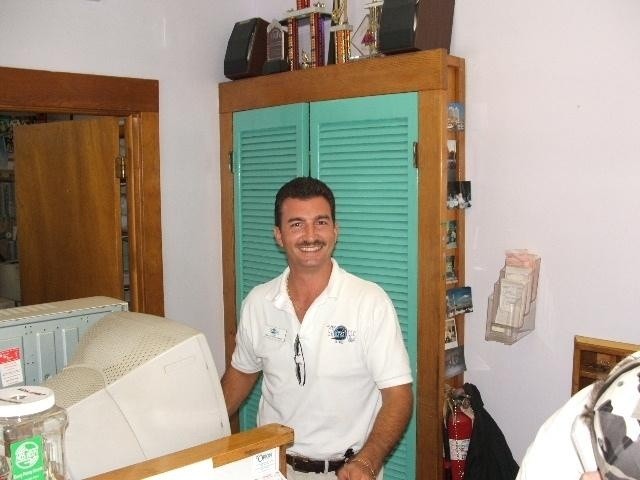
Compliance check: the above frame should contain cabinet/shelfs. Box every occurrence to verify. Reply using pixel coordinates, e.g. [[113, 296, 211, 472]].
[[216, 46, 466, 480]]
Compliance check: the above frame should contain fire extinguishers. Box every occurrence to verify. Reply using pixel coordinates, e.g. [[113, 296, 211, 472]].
[[448, 387, 473, 480]]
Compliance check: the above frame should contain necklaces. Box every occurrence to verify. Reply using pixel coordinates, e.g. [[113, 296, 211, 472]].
[[290, 297, 311, 314]]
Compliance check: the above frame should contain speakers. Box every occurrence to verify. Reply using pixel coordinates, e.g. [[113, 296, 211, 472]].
[[378, 0, 455, 56], [224, 18, 289, 80]]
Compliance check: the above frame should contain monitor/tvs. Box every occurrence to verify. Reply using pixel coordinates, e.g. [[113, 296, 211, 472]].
[[31, 311, 232, 480]]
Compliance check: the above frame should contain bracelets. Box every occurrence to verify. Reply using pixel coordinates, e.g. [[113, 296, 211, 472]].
[[348, 455, 377, 478]]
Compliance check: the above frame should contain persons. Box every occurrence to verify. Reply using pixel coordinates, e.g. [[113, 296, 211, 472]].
[[220, 174, 415, 479]]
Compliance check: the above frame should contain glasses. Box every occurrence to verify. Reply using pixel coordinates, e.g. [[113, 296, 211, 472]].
[[294, 334, 305, 387]]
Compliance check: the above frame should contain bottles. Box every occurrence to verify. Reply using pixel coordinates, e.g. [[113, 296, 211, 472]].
[[0, 386, 73, 480]]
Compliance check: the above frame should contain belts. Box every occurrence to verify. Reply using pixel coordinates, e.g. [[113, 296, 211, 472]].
[[286, 454, 345, 473]]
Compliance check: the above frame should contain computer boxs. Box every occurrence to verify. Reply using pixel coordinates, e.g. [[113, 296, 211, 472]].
[[0, 295, 128, 390]]
